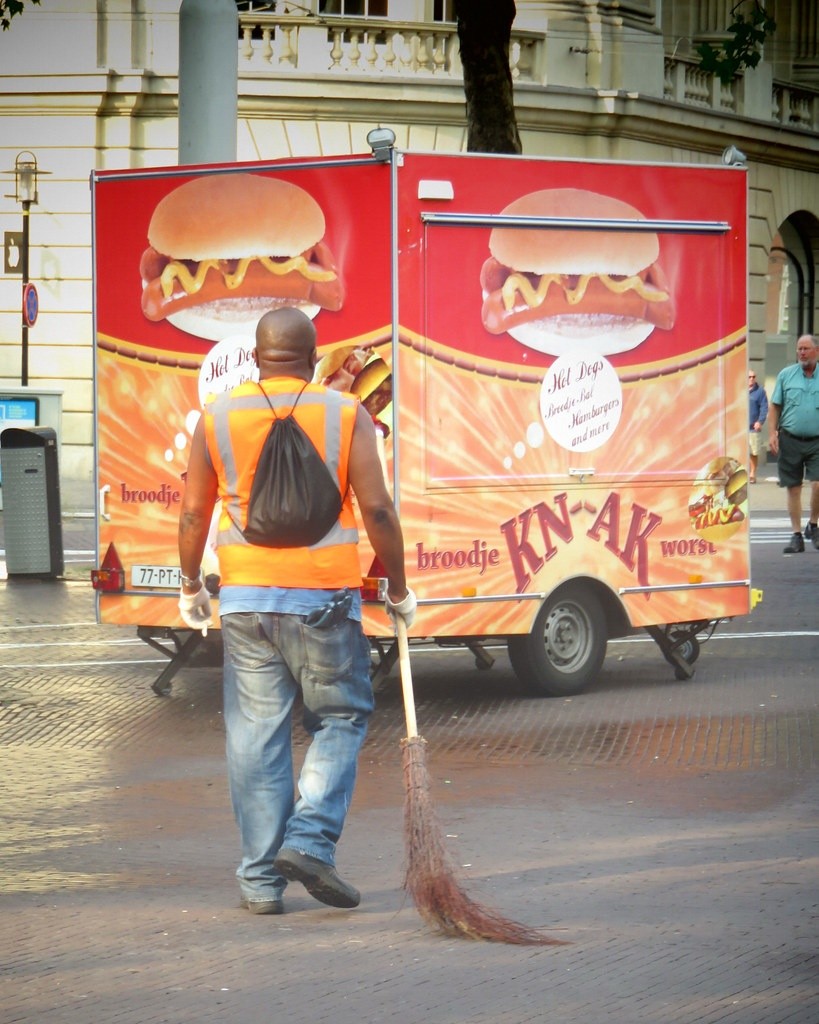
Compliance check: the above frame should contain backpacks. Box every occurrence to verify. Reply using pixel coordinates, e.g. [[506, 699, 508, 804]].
[[242, 383, 342, 549]]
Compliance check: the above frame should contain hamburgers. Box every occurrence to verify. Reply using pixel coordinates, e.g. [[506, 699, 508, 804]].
[[139, 173, 344, 344], [313, 346, 392, 420], [687, 456, 749, 543], [480, 188, 675, 358]]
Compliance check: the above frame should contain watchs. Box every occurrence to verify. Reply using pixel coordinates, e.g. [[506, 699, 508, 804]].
[[179, 568, 204, 587]]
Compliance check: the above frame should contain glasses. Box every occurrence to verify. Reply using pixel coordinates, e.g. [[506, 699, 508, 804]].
[[749, 375, 756, 378]]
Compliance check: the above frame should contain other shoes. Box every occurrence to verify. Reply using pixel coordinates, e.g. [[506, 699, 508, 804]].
[[750, 476, 756, 483], [240, 894, 283, 914], [274, 847, 360, 908]]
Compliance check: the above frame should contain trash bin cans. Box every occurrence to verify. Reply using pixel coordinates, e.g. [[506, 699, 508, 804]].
[[0, 427, 65, 580]]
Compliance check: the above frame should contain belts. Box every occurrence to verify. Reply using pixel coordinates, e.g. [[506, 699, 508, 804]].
[[780, 427, 818, 441]]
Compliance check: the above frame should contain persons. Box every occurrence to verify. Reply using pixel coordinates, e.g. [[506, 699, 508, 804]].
[[176, 305, 417, 916], [748, 369, 769, 483], [767, 333, 819, 554]]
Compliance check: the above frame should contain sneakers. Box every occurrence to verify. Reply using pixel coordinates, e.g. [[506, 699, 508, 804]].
[[803, 521, 819, 551], [782, 533, 804, 553]]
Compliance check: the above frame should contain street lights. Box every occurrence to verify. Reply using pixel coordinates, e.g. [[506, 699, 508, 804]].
[[15, 150, 38, 387]]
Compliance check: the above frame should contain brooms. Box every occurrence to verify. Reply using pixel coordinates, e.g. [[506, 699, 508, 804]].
[[372, 426, 569, 948]]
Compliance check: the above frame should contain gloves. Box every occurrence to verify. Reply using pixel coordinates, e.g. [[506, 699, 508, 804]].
[[384, 584, 416, 630], [177, 579, 213, 637]]
